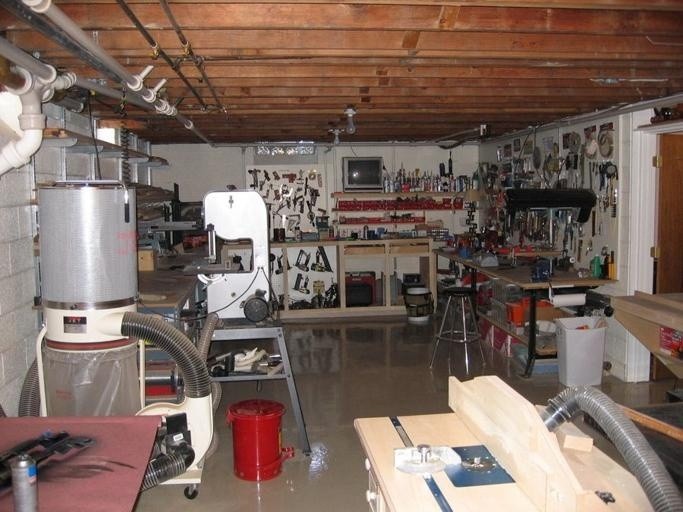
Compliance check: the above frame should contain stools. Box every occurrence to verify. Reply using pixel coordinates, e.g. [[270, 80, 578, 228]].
[[427, 286, 485, 377]]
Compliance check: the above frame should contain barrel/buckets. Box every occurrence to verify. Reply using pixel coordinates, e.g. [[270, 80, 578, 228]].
[[406, 286, 434, 323], [224, 399, 295, 482], [589, 254, 602, 279]]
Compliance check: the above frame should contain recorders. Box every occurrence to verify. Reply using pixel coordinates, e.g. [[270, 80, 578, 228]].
[[345, 270, 376, 307]]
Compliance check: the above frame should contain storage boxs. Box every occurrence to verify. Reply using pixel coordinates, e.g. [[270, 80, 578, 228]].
[[489, 279, 568, 326]]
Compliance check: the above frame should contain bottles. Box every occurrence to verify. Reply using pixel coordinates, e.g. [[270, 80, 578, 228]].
[[294, 226, 300, 242], [383, 163, 480, 193], [7, 455, 39, 512], [591, 251, 615, 280]]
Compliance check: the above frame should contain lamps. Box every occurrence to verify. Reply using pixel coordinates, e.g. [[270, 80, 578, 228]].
[[344, 108, 357, 134], [329, 129, 345, 146]]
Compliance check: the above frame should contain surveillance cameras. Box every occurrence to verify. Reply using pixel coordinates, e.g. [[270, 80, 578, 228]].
[[342, 155, 384, 193]]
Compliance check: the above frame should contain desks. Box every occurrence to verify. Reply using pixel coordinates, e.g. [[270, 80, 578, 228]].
[[137, 245, 208, 405], [0, 415, 162, 512], [432, 243, 620, 379], [354, 405, 656, 512]]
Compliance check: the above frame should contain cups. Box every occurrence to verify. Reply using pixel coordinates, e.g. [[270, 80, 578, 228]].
[[273, 228, 286, 241]]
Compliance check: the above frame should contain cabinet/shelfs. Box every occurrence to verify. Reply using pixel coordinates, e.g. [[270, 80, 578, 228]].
[[270, 238, 438, 318], [331, 191, 465, 225]]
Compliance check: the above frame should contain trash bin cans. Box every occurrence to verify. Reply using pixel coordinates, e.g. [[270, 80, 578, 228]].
[[555, 317, 607, 387]]
